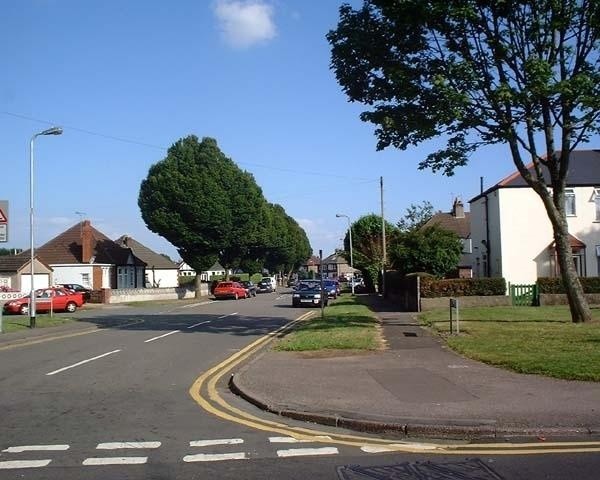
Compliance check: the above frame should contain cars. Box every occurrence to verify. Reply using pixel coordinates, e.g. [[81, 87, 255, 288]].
[[3, 282, 94, 314], [349, 278, 365, 289], [291, 279, 340, 308], [213, 277, 276, 299]]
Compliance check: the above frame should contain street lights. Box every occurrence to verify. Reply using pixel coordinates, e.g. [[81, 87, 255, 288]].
[[335, 213, 356, 296], [29, 126, 65, 329]]
